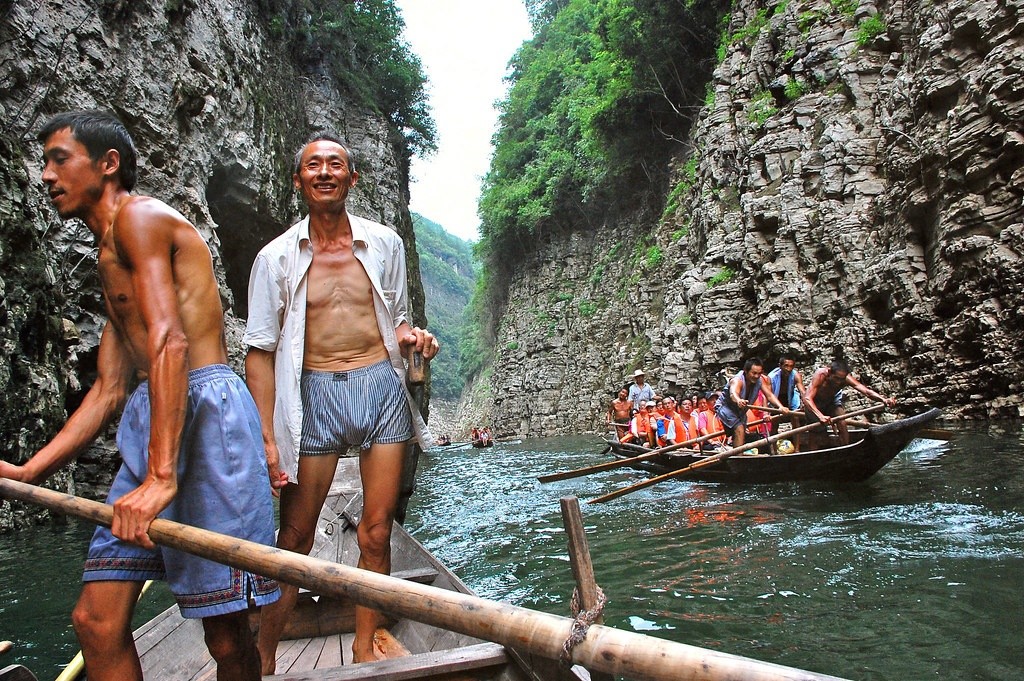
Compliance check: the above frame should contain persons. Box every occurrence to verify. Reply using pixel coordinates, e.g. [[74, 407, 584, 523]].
[[472, 426, 491, 446], [242, 133, 438, 676], [0, 111, 283, 681], [438, 435, 450, 442], [713, 355, 805, 456], [606, 369, 734, 455], [803, 363, 895, 452]]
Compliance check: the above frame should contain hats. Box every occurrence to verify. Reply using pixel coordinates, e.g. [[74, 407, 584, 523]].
[[633, 369, 645, 378], [645, 401, 656, 409], [705, 391, 718, 400], [629, 409, 639, 416]]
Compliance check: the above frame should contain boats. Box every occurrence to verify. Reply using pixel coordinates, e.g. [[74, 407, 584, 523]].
[[472, 439, 493, 448], [438, 441, 451, 446], [606, 407, 937, 490]]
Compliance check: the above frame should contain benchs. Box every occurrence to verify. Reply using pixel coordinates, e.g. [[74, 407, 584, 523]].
[[248, 567, 439, 642], [262, 642, 508, 681]]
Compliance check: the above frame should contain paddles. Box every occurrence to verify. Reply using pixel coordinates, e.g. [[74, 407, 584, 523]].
[[742, 401, 955, 442], [608, 422, 629, 427], [1, 478, 852, 681], [584, 398, 897, 505], [534, 402, 805, 486]]
